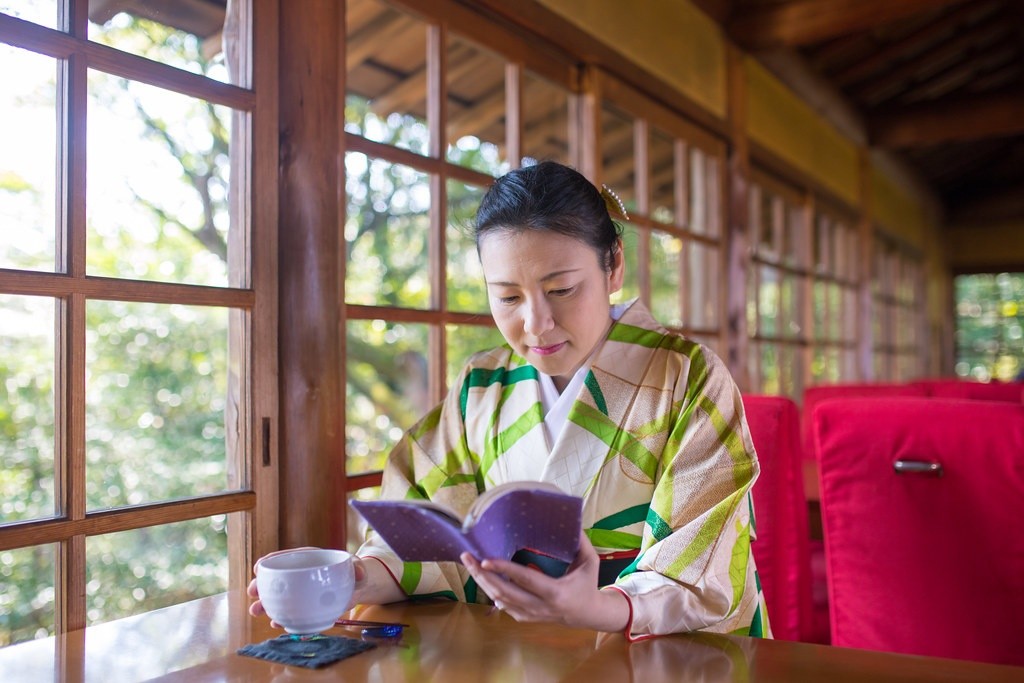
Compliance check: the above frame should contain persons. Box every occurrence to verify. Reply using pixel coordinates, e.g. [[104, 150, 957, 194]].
[[247, 160, 776, 642]]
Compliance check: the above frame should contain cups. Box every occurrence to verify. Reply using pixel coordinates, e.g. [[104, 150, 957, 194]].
[[256, 549, 356, 635]]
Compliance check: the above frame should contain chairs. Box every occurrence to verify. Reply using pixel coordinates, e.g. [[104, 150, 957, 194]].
[[740, 377, 1024, 667]]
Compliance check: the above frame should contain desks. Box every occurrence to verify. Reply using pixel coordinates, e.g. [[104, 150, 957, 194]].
[[0, 585, 1024, 683]]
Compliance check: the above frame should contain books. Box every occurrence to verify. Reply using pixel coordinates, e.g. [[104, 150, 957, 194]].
[[348, 480, 584, 573]]
